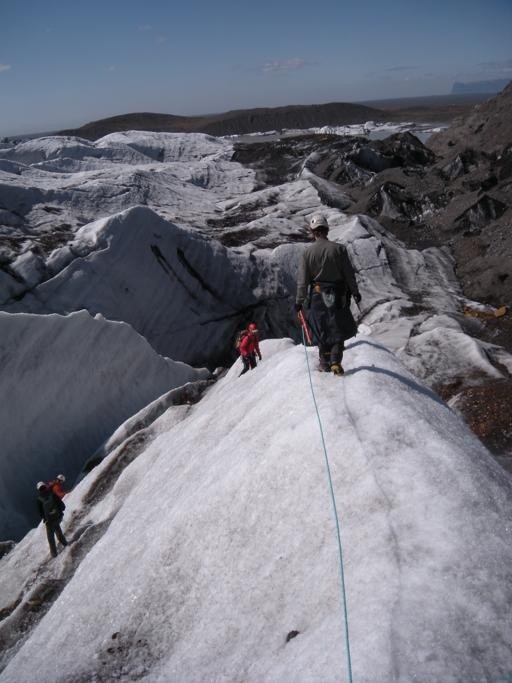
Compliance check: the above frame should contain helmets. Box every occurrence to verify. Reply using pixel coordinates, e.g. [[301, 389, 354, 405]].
[[308, 214, 329, 230]]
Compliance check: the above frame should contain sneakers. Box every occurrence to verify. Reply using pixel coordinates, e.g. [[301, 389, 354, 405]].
[[316, 360, 345, 375]]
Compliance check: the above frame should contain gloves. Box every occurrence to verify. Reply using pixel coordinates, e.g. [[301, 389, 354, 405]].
[[352, 292, 362, 303], [295, 303, 303, 312]]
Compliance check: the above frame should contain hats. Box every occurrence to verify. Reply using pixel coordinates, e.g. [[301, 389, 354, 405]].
[[248, 323, 260, 333]]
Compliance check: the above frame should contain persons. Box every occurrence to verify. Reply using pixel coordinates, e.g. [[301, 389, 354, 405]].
[[238, 323, 262, 376], [294, 215, 362, 377], [35, 480, 69, 558], [48, 474, 67, 499]]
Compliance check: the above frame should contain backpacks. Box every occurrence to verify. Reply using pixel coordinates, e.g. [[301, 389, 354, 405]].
[[37, 492, 62, 524], [234, 328, 248, 352]]
